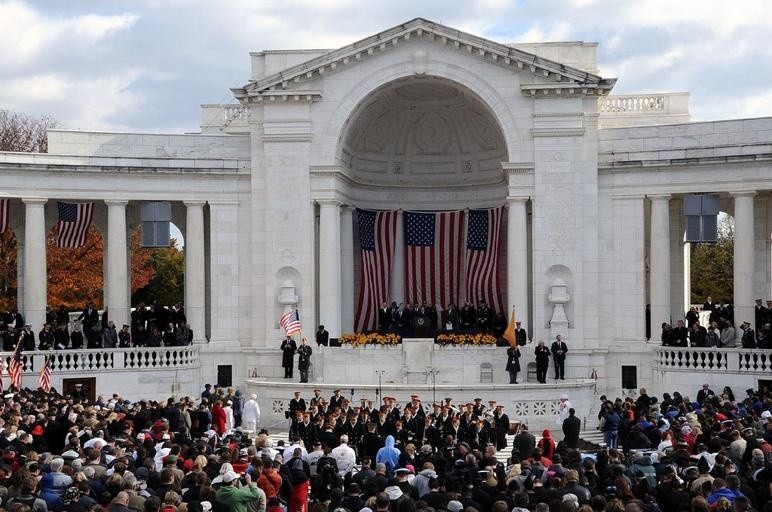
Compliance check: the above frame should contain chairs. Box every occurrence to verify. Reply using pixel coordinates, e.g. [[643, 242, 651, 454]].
[[527, 363, 537, 381], [303, 363, 313, 381], [481, 364, 493, 384]]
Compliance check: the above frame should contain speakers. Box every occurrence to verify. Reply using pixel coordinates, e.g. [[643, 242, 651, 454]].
[[217, 365, 232, 388], [683, 193, 720, 243], [141, 201, 172, 248], [63, 378, 96, 400], [621, 365, 638, 390]]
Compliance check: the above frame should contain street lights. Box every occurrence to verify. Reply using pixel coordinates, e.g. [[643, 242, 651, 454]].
[[430, 371, 439, 404], [376, 371, 385, 406]]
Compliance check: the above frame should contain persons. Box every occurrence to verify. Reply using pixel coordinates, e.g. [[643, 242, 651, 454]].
[[297, 336, 312, 382], [280, 334, 297, 378], [0, 379, 772, 512], [550, 335, 568, 380], [534, 340, 551, 383], [377, 300, 490, 336], [506, 343, 521, 383], [0, 295, 193, 354], [658, 294, 771, 372], [514, 321, 526, 346], [645, 304, 651, 340], [315, 323, 328, 347]]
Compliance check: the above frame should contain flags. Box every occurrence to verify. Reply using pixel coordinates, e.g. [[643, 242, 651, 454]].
[[39, 355, 54, 390], [403, 207, 466, 336], [278, 308, 302, 337], [8, 339, 25, 389], [502, 310, 519, 350], [466, 203, 507, 319], [352, 206, 398, 334], [0, 354, 3, 392]]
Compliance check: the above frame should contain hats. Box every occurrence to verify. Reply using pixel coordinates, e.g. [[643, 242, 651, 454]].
[[600, 386, 772, 492], [4, 386, 261, 512], [289, 388, 506, 430], [266, 430, 587, 512]]
